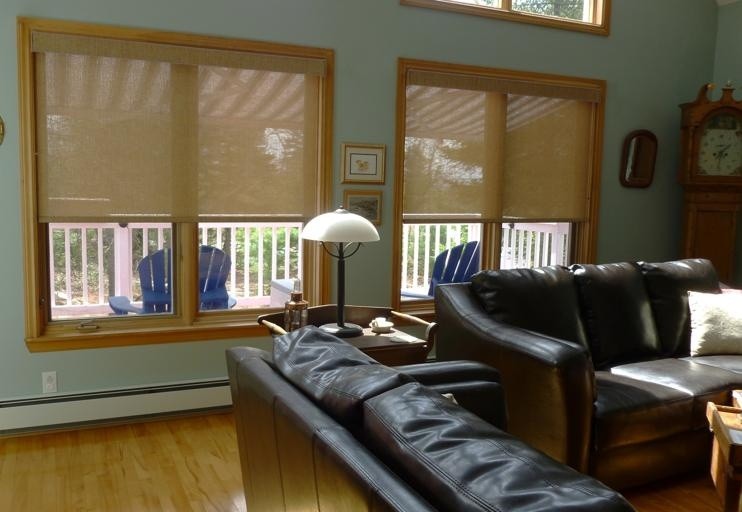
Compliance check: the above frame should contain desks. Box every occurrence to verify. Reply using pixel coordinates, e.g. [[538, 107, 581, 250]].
[[699, 389, 742, 512], [268, 278, 299, 306]]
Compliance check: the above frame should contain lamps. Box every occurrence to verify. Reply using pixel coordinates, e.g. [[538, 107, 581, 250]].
[[299, 207, 383, 338]]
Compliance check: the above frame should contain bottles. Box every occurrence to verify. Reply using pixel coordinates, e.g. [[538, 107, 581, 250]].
[[284, 279, 309, 331]]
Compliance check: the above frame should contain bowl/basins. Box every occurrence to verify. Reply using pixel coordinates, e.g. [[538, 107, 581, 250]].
[[368, 322, 394, 333]]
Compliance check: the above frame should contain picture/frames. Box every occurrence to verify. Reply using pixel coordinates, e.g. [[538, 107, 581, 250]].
[[340, 142, 387, 184], [342, 189, 384, 228]]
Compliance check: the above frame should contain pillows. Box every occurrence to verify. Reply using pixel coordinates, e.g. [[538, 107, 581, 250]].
[[685, 288, 741, 357]]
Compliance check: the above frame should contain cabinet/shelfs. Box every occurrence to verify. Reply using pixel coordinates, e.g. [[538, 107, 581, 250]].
[[256, 303, 437, 367]]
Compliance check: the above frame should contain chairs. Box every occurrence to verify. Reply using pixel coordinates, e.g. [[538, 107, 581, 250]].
[[105, 245, 236, 315], [402, 241, 479, 300]]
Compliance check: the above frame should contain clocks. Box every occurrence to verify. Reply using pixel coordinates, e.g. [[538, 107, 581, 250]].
[[676, 78, 742, 286]]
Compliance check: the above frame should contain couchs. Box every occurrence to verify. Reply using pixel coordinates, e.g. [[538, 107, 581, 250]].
[[217, 325, 649, 512], [428, 256, 742, 488]]
